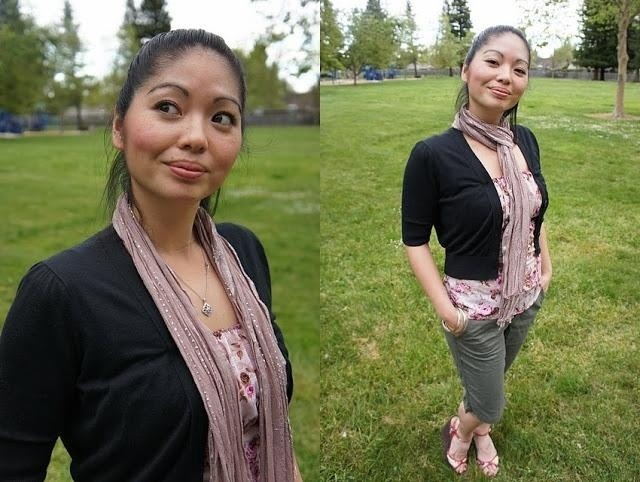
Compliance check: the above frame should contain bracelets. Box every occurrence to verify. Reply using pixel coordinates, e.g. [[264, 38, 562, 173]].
[[435, 307, 470, 338]]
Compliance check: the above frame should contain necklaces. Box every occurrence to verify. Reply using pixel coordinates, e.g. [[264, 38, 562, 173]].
[[161, 240, 215, 320]]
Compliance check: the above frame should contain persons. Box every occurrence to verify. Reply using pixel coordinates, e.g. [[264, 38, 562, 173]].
[[1, 27, 306, 482], [393, 24, 555, 476]]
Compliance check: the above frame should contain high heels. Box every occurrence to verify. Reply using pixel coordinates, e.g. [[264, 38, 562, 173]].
[[473, 427, 499, 477], [443, 416, 473, 474]]
